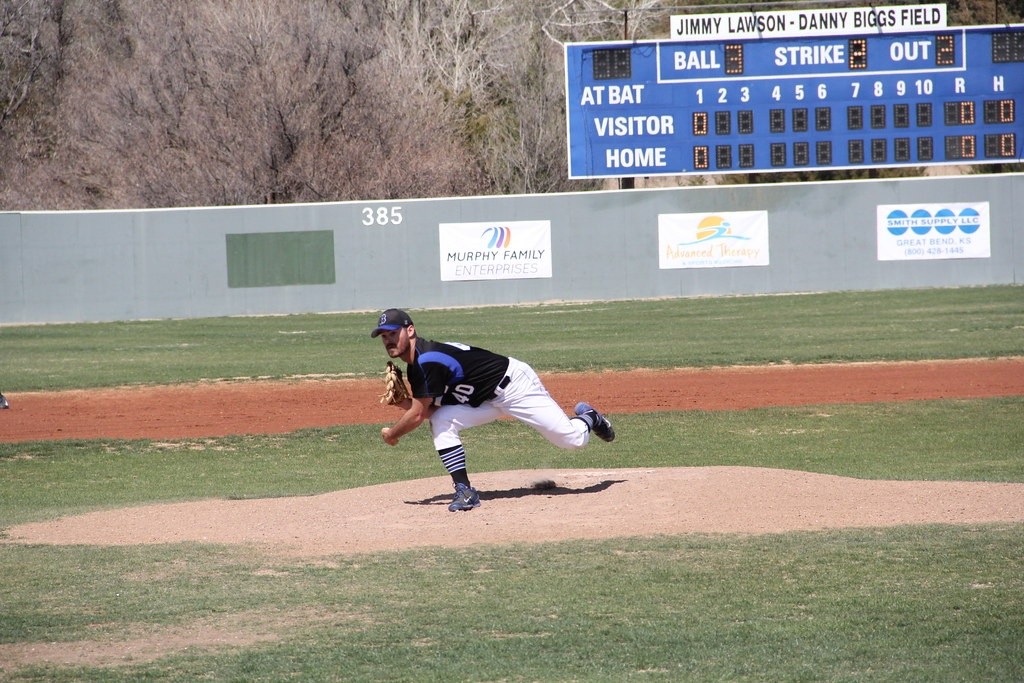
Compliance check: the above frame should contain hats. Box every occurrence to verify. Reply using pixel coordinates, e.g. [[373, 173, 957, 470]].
[[371, 308, 412, 338]]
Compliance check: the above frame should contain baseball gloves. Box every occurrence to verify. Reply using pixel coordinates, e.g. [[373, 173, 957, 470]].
[[385, 361, 411, 405]]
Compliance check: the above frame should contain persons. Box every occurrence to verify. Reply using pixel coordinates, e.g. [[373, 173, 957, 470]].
[[371, 308, 615, 512]]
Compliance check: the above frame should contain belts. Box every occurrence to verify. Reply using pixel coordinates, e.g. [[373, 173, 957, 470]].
[[489, 376, 510, 401]]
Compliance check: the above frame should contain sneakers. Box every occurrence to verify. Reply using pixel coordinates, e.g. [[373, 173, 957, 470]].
[[574, 402, 615, 442], [448, 482, 480, 512]]
